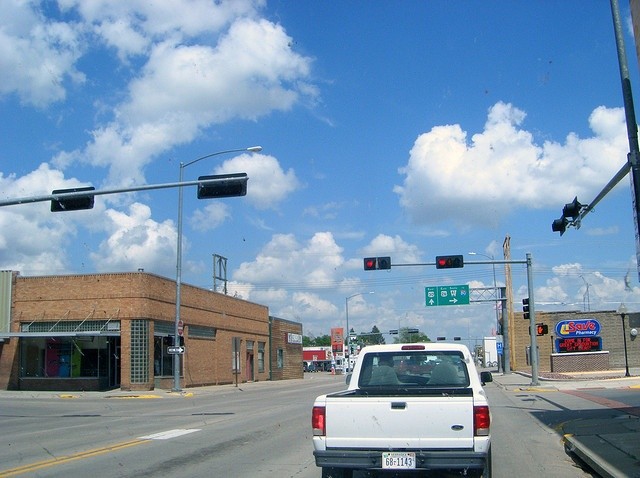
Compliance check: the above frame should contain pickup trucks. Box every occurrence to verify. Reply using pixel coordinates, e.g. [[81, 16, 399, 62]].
[[312, 343, 492, 478]]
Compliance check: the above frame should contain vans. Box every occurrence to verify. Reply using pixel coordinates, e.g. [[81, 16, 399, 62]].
[[309, 361, 331, 371]]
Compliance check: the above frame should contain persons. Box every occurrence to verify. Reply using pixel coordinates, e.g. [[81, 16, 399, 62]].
[[377, 357, 397, 368]]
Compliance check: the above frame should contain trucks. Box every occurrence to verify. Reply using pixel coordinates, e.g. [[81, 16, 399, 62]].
[[326, 359, 353, 372]]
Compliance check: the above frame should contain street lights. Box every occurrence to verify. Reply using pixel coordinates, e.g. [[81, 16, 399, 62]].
[[172, 146, 262, 392], [616, 303, 631, 376], [468, 252, 502, 372], [346, 291, 375, 372]]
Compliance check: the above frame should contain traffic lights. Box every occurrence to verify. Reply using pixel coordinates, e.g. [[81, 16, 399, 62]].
[[538, 325, 548, 334], [522, 298, 529, 319], [364, 257, 391, 269], [454, 337, 461, 340], [437, 337, 445, 340], [436, 255, 463, 269]]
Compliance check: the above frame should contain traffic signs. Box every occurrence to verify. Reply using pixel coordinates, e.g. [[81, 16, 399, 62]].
[[425, 285, 469, 306]]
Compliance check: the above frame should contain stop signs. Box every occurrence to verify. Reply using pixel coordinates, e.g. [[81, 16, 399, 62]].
[[178, 321, 183, 335]]
